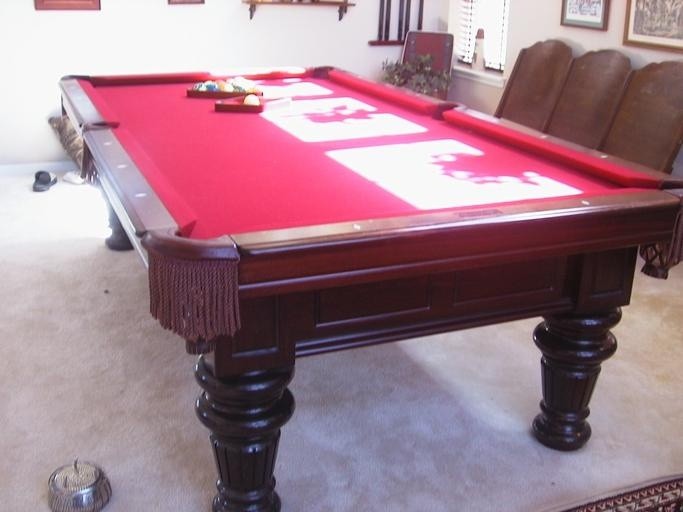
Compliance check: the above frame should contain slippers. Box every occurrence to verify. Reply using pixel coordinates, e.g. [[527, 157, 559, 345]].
[[34, 170, 57, 191]]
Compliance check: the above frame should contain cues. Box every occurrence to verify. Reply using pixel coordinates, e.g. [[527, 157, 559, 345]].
[[378, 1, 423, 43]]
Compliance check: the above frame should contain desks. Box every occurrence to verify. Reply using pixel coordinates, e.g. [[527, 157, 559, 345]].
[[58, 65, 683, 512]]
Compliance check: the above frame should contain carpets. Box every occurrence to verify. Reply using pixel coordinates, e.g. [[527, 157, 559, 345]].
[[536, 472, 682, 511]]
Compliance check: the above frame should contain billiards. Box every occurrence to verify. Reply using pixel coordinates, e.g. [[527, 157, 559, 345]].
[[243, 94, 259, 105], [193, 77, 259, 93]]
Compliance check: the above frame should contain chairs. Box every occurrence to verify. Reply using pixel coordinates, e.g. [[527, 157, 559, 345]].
[[394, 30, 454, 101], [493, 40, 683, 174]]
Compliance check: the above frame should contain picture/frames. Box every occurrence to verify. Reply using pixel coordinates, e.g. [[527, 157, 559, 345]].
[[557, 0, 683, 55]]
